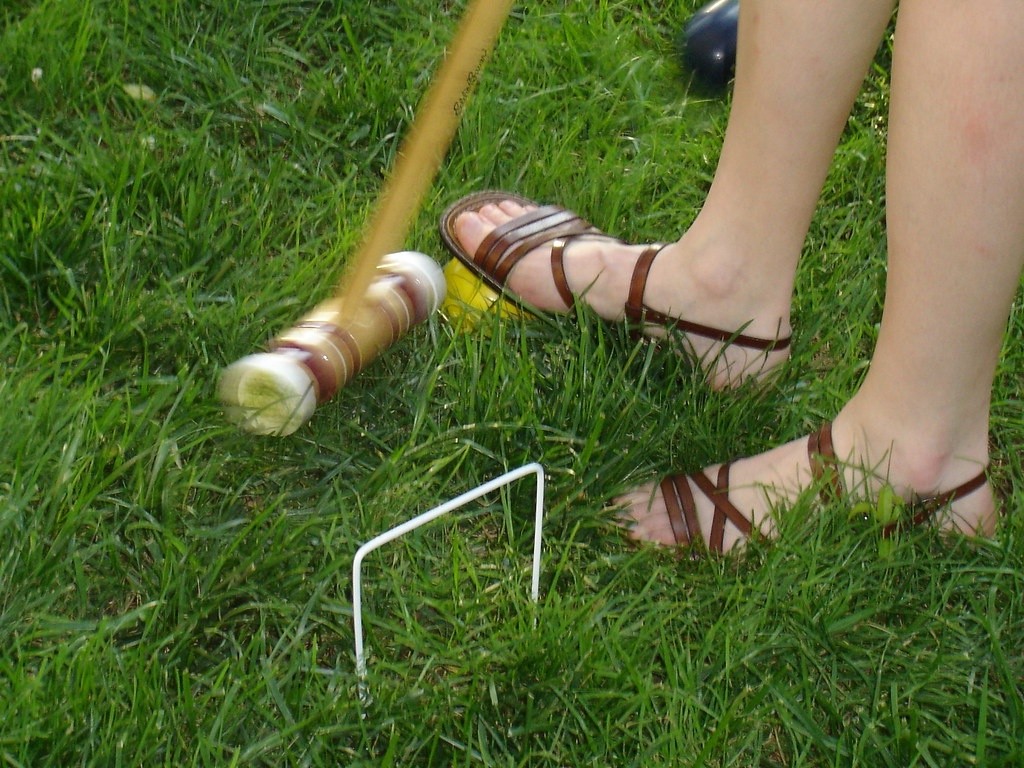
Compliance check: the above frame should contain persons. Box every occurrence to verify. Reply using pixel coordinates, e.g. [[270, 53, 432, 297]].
[[439, 1, 1024, 575]]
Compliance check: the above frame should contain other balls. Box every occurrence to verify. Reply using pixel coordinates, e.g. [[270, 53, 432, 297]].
[[443, 254, 536, 331], [681, 1, 742, 97]]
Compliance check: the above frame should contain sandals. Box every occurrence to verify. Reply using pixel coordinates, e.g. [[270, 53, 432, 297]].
[[439, 190, 793, 396], [608, 421, 997, 567]]
